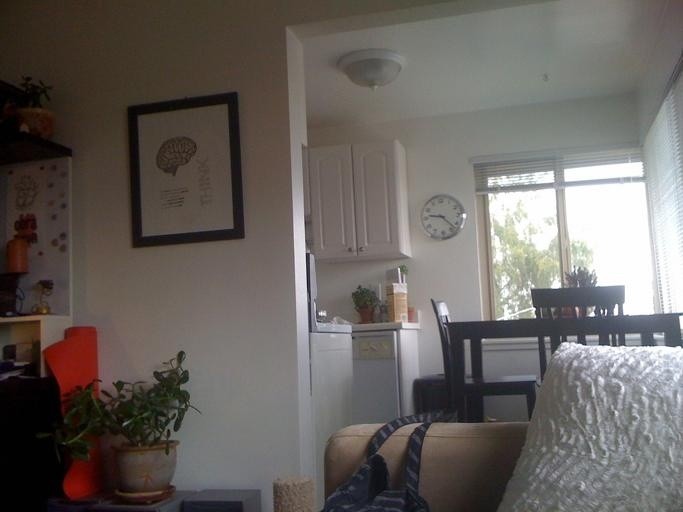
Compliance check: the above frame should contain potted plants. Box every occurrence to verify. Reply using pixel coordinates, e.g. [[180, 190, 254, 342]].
[[38, 352, 203, 502], [565, 265, 598, 316], [351, 286, 380, 323], [14, 74, 55, 141]]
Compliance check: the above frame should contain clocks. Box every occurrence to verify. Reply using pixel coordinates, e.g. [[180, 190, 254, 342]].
[[420, 194, 467, 241]]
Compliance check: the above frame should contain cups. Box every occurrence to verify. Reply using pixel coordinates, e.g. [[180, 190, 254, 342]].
[[378, 300, 387, 323], [315, 309, 326, 323], [407, 308, 415, 322]]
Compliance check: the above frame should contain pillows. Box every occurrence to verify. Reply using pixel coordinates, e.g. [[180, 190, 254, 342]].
[[494, 342, 683, 512]]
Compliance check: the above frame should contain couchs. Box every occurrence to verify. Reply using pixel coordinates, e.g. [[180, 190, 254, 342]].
[[323, 421, 528, 511]]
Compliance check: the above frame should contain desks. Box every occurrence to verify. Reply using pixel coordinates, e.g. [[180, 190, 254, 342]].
[[448, 312, 683, 422], [0, 314, 73, 378]]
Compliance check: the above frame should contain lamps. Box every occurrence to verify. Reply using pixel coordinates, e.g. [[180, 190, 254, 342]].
[[337, 48, 405, 91]]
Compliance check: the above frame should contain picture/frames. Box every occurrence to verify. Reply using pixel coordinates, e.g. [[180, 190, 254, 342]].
[[126, 92, 245, 249]]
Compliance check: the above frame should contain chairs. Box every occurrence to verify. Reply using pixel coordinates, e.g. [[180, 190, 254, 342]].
[[430, 298, 537, 423], [530, 285, 625, 385]]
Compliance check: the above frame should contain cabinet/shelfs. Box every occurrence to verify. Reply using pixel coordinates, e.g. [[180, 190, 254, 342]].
[[352, 321, 423, 423], [309, 139, 413, 264]]
